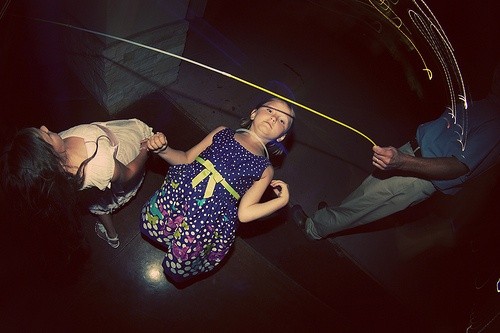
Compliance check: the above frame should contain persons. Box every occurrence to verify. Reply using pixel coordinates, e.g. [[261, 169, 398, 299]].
[[291, 85, 500, 243], [138, 95, 297, 285], [4, 119, 155, 248]]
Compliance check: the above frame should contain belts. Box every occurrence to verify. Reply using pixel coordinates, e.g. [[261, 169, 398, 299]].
[[409, 137, 422, 158]]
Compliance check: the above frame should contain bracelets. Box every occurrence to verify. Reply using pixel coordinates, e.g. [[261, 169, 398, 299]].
[[139, 147, 150, 152]]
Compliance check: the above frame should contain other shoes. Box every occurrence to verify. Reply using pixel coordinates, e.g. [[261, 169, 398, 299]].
[[291, 204, 311, 240], [318, 201, 328, 211]]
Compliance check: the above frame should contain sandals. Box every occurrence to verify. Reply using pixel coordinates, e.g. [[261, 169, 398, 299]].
[[94, 219, 120, 248]]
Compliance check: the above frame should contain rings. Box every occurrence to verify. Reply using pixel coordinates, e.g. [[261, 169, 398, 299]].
[[375, 158, 378, 162]]
